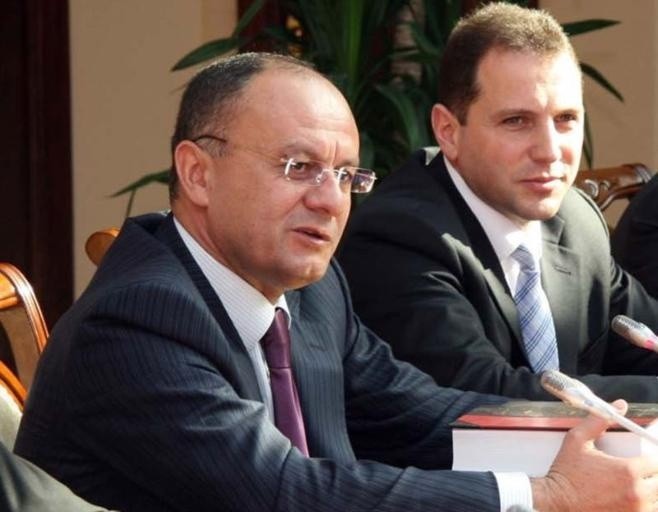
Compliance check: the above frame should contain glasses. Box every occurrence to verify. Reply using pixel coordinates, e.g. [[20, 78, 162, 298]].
[[192, 134, 378, 194]]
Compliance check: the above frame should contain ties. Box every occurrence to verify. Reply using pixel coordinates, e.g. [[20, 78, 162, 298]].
[[262, 309, 311, 459], [511, 245, 560, 375]]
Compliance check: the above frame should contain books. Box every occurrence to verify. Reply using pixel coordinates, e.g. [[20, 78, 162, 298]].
[[447, 400, 658, 478]]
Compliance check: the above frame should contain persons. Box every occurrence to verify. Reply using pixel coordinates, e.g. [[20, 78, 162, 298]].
[[10, 53, 658, 510], [332, 1, 658, 402]]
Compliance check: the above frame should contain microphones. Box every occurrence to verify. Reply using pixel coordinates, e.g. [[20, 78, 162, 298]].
[[540, 369, 658, 445], [611, 314, 658, 352]]
